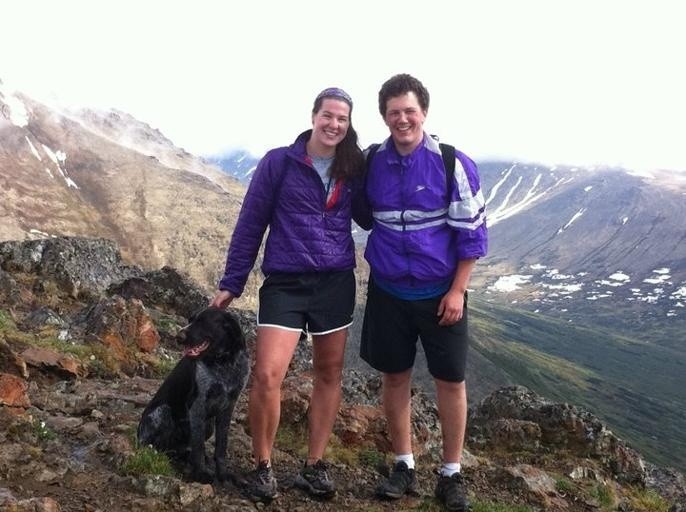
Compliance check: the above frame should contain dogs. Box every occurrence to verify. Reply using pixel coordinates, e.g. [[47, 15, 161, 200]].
[[137, 306, 252, 490]]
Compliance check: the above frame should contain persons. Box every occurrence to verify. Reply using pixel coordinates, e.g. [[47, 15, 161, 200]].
[[359, 74, 489, 512], [208, 87, 374, 499]]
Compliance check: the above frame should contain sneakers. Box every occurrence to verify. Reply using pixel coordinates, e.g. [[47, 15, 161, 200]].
[[433, 466, 469, 512], [253, 456, 277, 499], [295, 458, 337, 498], [375, 459, 417, 499]]
